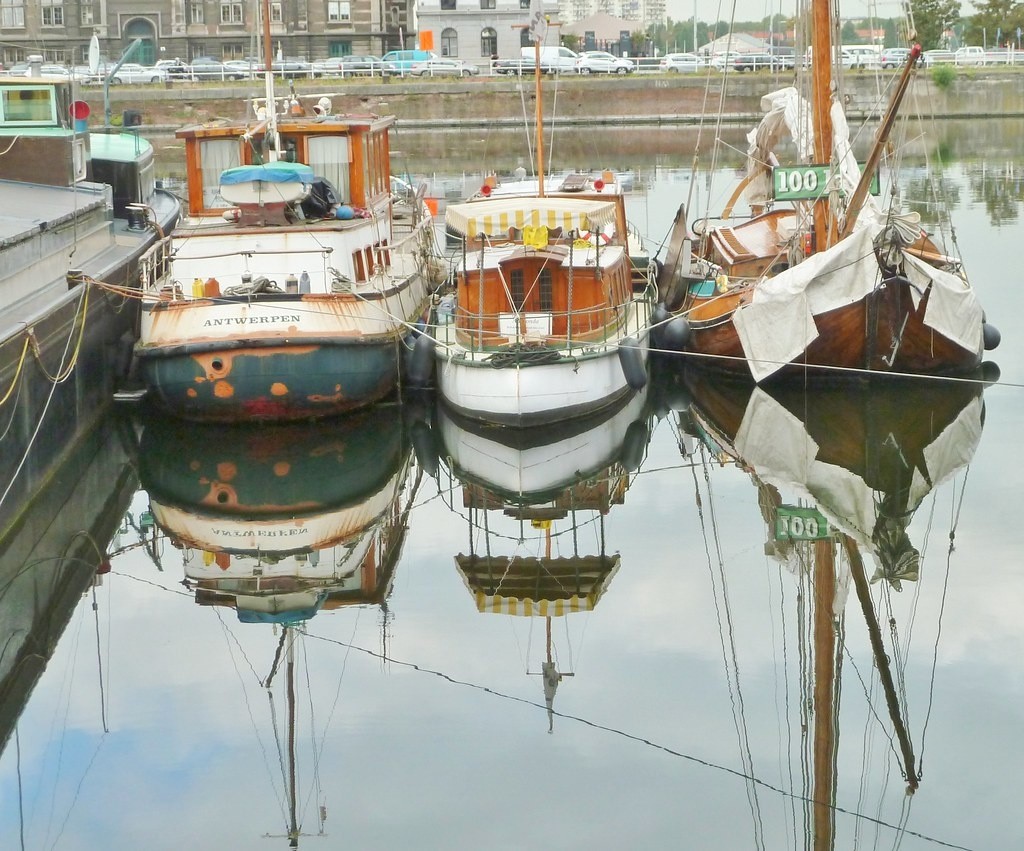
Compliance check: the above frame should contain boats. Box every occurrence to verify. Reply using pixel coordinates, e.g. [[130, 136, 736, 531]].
[[421, 370, 655, 735], [134, 383, 423, 851], [0, 312, 144, 757], [432, 17, 657, 429], [137, 2, 444, 424], [0, 55, 182, 422]]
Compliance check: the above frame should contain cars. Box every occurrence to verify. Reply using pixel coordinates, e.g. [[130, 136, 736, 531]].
[[954, 46, 987, 66], [846, 47, 882, 66], [1, 51, 479, 85], [710, 51, 742, 70], [494, 56, 557, 76], [733, 53, 790, 72], [878, 47, 934, 69], [659, 54, 721, 73], [988, 48, 1020, 67]]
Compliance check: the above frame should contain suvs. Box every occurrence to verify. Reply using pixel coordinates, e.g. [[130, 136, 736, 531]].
[[575, 52, 636, 76]]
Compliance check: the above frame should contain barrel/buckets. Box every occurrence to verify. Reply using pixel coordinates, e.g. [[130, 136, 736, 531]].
[[69, 100, 90, 119], [75, 120, 87, 132], [485, 176, 496, 188], [594, 180, 605, 190], [482, 185, 492, 195], [601, 170, 614, 183]]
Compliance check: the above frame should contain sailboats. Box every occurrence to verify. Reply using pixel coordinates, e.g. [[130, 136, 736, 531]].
[[663, 366, 985, 850], [653, 0, 986, 377]]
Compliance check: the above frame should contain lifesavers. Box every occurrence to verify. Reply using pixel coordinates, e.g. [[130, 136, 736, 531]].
[[578, 216, 615, 244], [801, 233, 812, 255]]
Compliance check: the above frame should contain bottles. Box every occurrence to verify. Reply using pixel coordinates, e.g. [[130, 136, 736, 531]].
[[300, 270, 311, 293], [205, 278, 219, 297], [285, 274, 298, 293], [192, 278, 204, 298]]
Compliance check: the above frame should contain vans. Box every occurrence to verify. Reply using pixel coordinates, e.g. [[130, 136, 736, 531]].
[[805, 46, 865, 72], [521, 46, 579, 73]]
